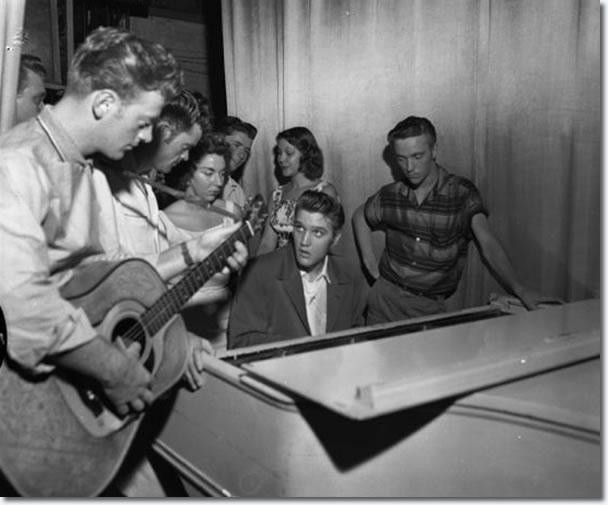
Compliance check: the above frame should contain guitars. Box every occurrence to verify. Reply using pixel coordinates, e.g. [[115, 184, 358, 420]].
[[0, 194, 269, 498]]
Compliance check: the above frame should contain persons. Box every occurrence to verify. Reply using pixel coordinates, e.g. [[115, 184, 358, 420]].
[[351, 115, 567, 325], [0, 25, 249, 497], [164, 133, 242, 340], [213, 116, 258, 175], [256, 126, 339, 255], [85, 89, 213, 254], [15, 54, 49, 127], [226, 190, 372, 351]]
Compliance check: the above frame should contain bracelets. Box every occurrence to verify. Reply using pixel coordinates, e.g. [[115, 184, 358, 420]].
[[180, 241, 194, 268]]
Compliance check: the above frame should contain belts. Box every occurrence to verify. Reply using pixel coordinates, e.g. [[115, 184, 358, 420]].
[[381, 277, 449, 301]]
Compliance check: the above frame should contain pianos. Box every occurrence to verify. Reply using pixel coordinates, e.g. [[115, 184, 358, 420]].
[[139, 292, 603, 499]]
